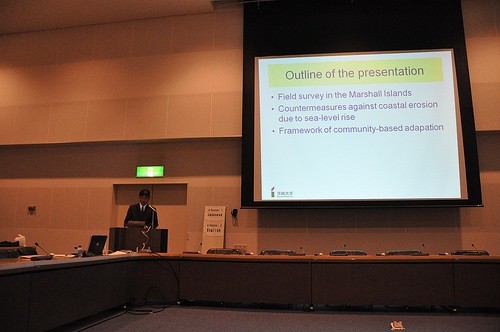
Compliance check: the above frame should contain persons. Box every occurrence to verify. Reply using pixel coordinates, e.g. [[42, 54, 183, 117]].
[[124, 189, 158, 229]]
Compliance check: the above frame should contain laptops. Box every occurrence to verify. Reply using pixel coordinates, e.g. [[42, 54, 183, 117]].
[[87, 234, 108, 255]]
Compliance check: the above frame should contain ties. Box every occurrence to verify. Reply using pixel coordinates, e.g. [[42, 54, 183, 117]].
[[142, 207, 143, 212]]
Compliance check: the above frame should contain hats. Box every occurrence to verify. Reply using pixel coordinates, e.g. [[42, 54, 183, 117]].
[[139, 189, 150, 197]]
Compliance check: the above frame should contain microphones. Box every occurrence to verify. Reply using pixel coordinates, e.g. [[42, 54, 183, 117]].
[[198, 243, 202, 251], [472, 243, 481, 254], [423, 244, 424, 246], [344, 243, 348, 255], [300, 246, 305, 254], [35, 242, 49, 254]]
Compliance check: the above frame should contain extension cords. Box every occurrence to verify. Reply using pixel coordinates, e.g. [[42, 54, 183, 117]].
[[140, 249, 152, 252]]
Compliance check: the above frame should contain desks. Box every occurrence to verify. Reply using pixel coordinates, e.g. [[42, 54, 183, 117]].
[[0, 253, 500, 332]]
